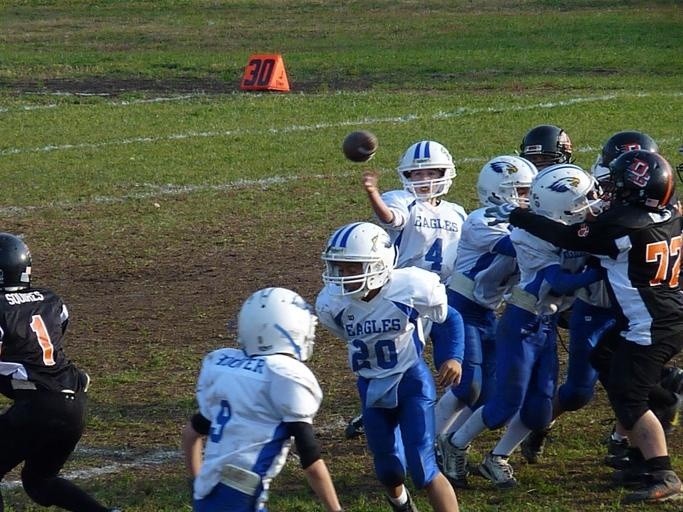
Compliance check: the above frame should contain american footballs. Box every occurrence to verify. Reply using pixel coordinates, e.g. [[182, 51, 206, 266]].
[[344, 131, 377, 161]]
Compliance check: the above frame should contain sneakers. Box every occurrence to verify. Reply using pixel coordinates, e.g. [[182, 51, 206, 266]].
[[602, 425, 683, 503], [345, 414, 364, 440], [435, 430, 548, 487]]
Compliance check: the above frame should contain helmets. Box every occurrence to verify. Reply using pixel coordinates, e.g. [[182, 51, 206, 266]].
[[322, 223, 395, 300], [1, 232, 33, 290], [398, 141, 456, 203], [238, 287, 319, 362], [476, 126, 675, 227]]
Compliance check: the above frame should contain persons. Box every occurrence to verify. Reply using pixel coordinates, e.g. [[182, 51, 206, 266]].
[[0, 231, 114, 512], [317, 126, 682, 512], [184, 288, 342, 512]]
